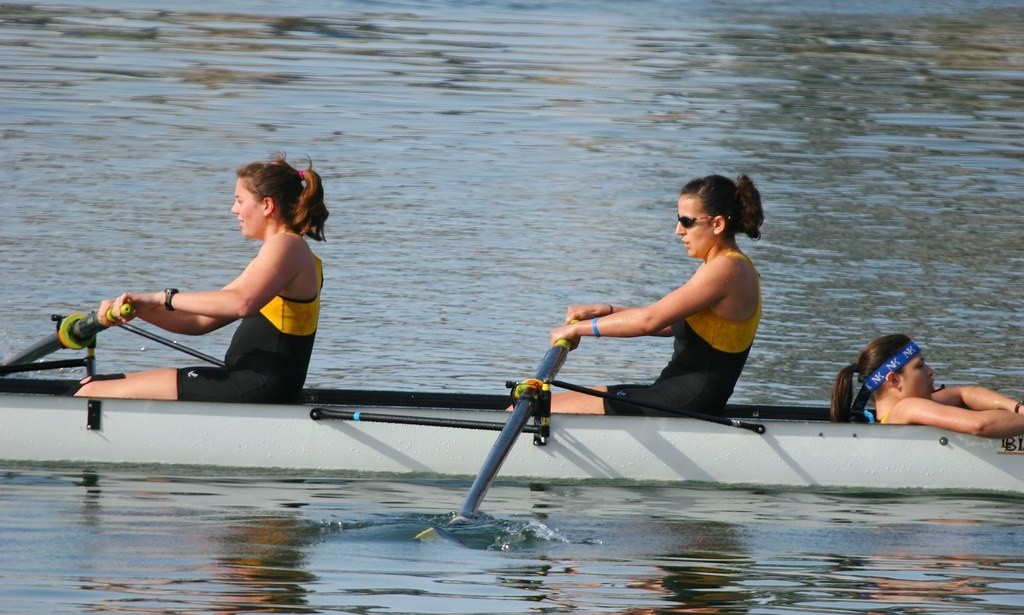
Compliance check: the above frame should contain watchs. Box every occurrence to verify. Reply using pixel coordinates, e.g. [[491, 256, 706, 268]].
[[163, 288, 179, 312]]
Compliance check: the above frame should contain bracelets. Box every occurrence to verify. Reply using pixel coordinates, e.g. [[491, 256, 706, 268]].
[[609, 304, 614, 314], [592, 317, 601, 338], [1015, 401, 1024, 413]]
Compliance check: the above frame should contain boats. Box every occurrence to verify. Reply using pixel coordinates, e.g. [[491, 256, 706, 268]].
[[0, 365, 1024, 506]]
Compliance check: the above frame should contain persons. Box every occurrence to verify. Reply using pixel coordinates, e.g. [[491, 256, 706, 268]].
[[817, 332, 1024, 447], [74, 152, 329, 401], [501, 172, 764, 419]]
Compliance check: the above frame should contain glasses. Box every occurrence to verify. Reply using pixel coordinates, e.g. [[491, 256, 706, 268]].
[[677, 214, 714, 229]]
